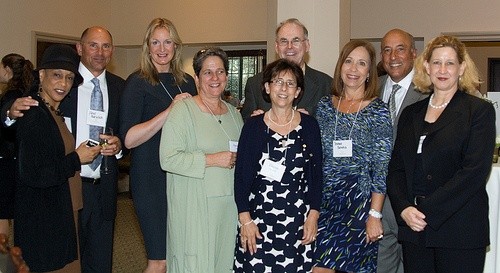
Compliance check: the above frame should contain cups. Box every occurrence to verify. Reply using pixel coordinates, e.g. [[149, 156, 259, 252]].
[[96, 127, 113, 152]]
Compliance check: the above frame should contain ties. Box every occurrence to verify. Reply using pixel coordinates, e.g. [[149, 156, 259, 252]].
[[388, 85, 402, 127], [88, 78, 104, 171]]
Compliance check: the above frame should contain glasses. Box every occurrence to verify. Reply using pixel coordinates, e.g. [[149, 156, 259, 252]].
[[275, 38, 307, 46], [268, 79, 297, 88]]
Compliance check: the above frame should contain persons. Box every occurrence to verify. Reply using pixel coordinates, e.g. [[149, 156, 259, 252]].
[[242, 19, 334, 116], [0, 25, 129, 273], [375, 29, 428, 273], [118, 18, 198, 273], [387, 35, 496, 273], [233, 59, 322, 273], [160, 48, 245, 273], [311, 40, 393, 273]]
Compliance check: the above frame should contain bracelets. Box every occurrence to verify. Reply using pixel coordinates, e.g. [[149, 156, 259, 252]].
[[238, 219, 253, 226], [368, 209, 382, 218]]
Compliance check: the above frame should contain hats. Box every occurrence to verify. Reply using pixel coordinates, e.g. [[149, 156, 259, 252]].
[[33, 44, 83, 87]]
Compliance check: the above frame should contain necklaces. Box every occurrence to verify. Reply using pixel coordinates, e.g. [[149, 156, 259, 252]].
[[268, 108, 294, 126], [347, 102, 354, 113], [159, 77, 182, 100], [218, 106, 222, 124], [429, 94, 451, 108], [37, 93, 65, 123]]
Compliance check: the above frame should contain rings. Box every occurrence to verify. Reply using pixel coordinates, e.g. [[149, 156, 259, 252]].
[[313, 237, 315, 240], [230, 165, 233, 168], [113, 148, 116, 152], [378, 235, 383, 238]]
